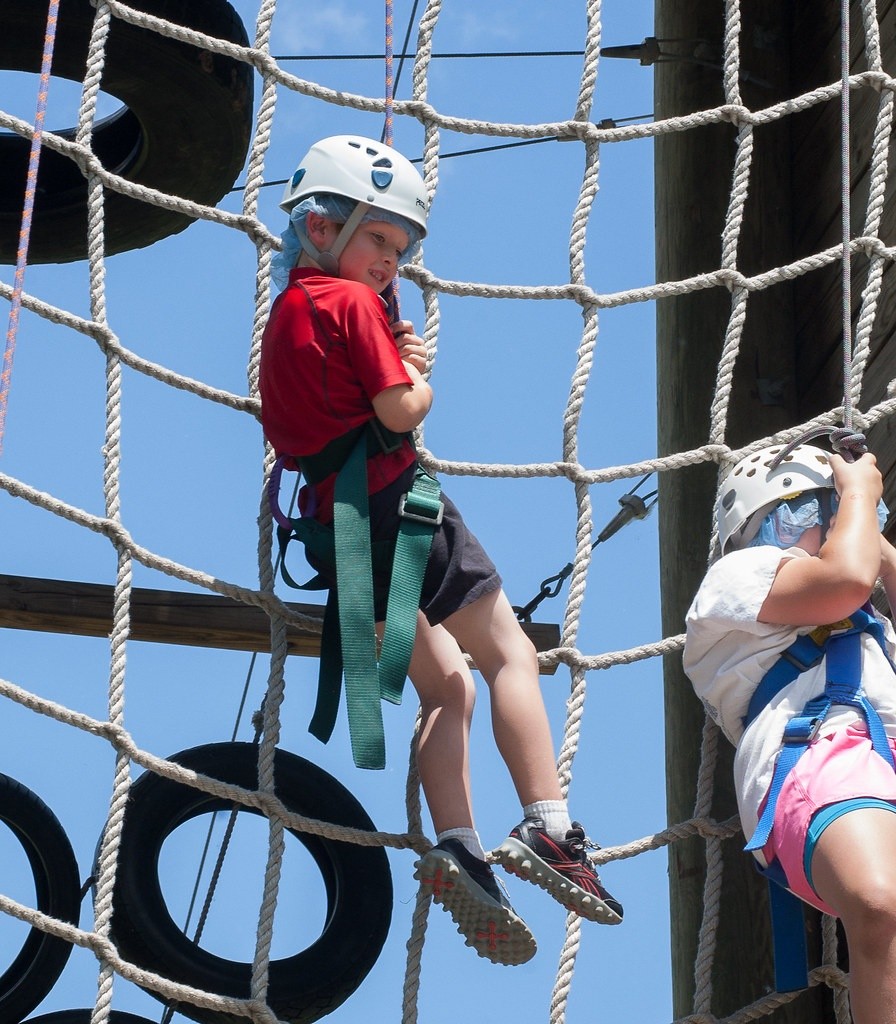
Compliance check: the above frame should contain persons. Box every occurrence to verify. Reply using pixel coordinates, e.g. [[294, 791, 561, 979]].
[[260, 132, 624, 967], [681, 438, 896, 1023]]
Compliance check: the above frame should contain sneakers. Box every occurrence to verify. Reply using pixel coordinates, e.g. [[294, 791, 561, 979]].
[[491, 817, 624, 926], [412, 836, 538, 966]]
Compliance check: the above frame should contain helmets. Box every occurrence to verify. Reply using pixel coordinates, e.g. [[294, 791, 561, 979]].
[[719, 444, 835, 556], [279, 134, 428, 239]]
